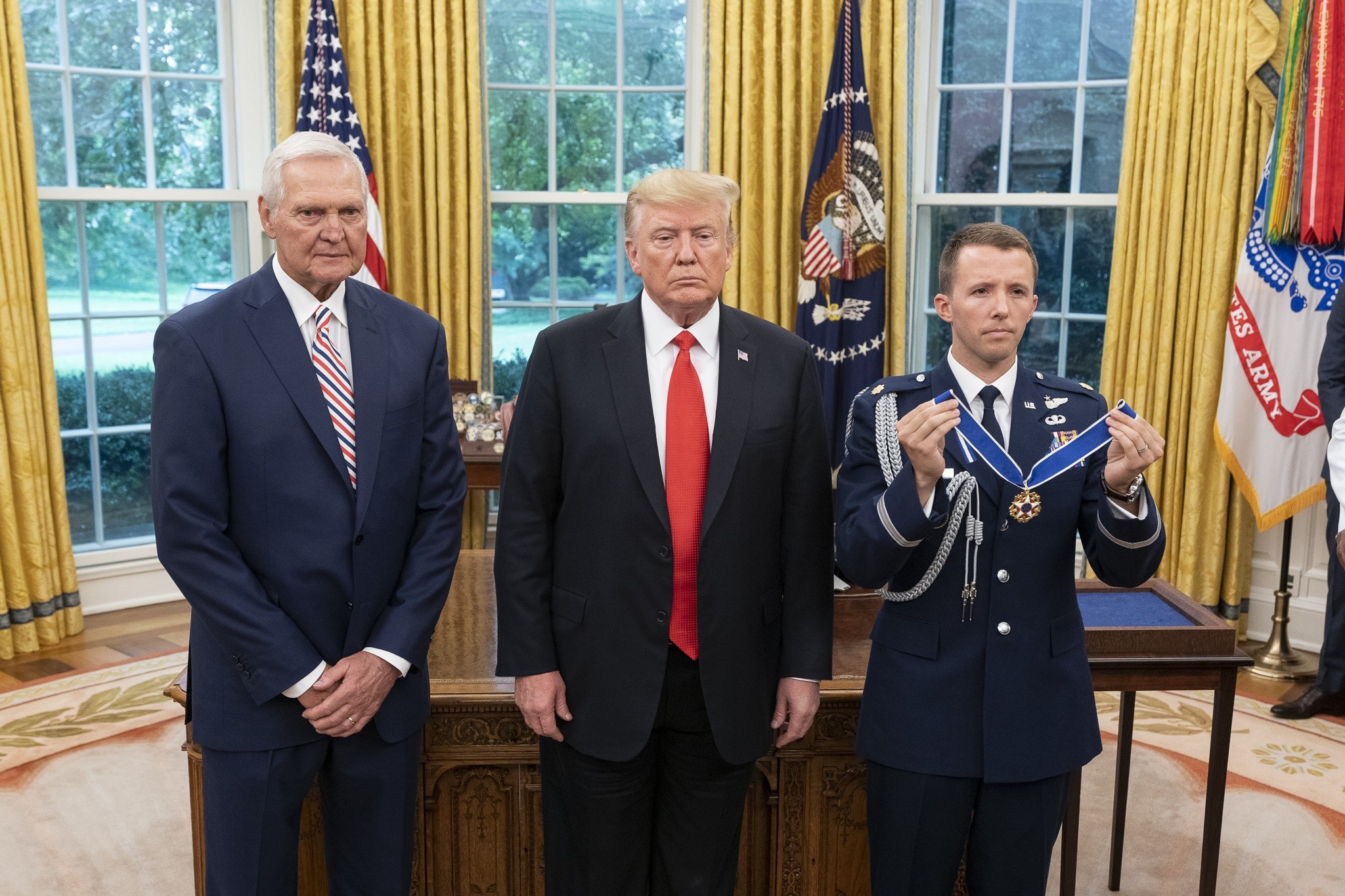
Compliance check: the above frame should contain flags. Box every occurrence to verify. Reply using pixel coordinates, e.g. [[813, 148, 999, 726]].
[[290, 1, 389, 294], [1213, 0, 1345, 533], [795, 0, 889, 594]]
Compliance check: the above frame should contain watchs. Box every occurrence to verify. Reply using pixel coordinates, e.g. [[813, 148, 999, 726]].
[[1099, 465, 1146, 504]]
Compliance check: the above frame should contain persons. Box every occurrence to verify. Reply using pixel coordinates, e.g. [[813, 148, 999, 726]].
[[1269, 281, 1345, 722], [492, 167, 841, 896], [145, 130, 469, 896], [833, 222, 1167, 896]]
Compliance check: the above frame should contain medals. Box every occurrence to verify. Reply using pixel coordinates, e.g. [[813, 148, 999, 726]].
[[1007, 488, 1043, 525]]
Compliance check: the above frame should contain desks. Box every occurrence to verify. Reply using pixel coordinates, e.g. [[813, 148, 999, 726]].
[[164, 549, 885, 896]]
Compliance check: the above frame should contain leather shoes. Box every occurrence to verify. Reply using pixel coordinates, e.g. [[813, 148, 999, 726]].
[[1270, 688, 1345, 719]]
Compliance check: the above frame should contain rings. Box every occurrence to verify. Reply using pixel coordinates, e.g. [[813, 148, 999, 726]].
[[1136, 443, 1151, 454], [347, 717, 356, 726]]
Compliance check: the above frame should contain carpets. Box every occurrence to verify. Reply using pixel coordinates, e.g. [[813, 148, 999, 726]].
[[0, 646, 195, 896], [1046, 683, 1345, 896]]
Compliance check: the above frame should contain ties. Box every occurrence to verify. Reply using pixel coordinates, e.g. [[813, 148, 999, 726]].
[[665, 332, 712, 661], [311, 304, 356, 491], [977, 385, 1006, 453]]
